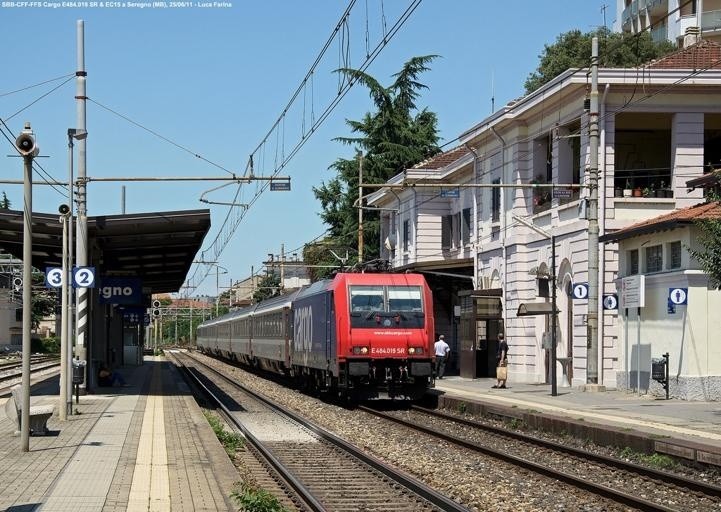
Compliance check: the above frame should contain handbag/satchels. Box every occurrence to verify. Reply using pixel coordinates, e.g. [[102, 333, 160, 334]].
[[497, 367, 507, 380]]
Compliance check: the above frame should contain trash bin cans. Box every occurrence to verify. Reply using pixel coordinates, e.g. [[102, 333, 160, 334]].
[[652, 352, 669, 399], [72, 356, 84, 403]]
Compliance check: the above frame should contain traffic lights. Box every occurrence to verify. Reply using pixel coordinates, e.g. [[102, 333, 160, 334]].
[[153, 299, 162, 317]]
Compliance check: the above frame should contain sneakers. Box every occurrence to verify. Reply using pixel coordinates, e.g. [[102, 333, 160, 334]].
[[491, 384, 506, 388]]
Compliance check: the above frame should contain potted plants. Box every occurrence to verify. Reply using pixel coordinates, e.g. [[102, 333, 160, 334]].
[[623, 179, 673, 198]]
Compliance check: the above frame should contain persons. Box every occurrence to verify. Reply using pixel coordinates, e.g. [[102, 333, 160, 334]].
[[435, 335, 451, 380], [491, 332, 508, 389]]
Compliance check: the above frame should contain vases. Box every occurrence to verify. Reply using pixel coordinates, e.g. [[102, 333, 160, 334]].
[[614, 188, 623, 197]]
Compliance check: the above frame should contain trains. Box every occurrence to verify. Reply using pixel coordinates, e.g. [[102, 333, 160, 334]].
[[196, 271, 438, 408]]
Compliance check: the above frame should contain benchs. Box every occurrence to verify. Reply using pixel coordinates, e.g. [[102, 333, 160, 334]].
[[10, 384, 57, 438]]
